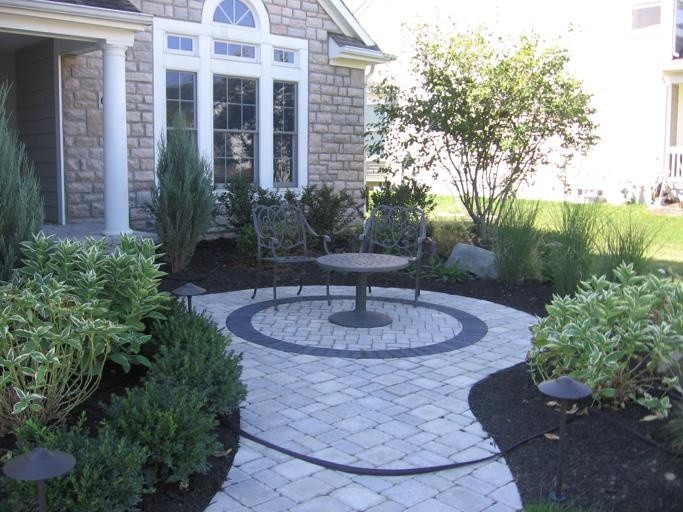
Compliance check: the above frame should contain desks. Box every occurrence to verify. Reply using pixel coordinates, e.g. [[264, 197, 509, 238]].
[[317, 252, 409, 329]]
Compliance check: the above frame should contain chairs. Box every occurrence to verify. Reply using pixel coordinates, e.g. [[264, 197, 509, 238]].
[[250, 202, 333, 311], [358, 204, 427, 308]]
